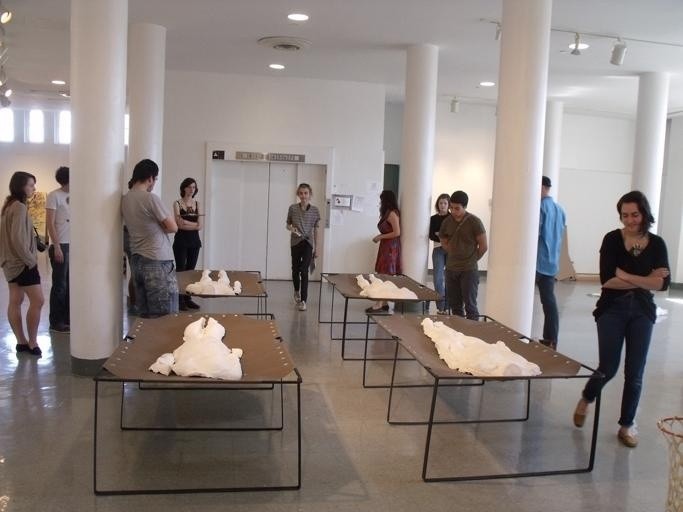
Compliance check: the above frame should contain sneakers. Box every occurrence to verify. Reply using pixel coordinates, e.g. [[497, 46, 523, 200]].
[[298, 301, 306, 311], [294, 291, 301, 303]]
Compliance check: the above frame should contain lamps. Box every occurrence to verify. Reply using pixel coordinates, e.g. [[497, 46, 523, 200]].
[[570, 33, 580, 55], [450, 97, 459, 113], [610, 37, 627, 65], [0, 4, 13, 108]]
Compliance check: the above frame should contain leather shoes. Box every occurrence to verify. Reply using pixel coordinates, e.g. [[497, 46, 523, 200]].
[[617, 429, 636, 447], [17, 344, 28, 352], [49, 322, 70, 334], [574, 399, 587, 427], [26, 345, 41, 355]]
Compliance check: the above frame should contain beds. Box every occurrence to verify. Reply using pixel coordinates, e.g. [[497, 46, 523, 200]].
[[172, 269, 270, 320], [317, 272, 444, 361], [362, 310, 606, 483], [91, 311, 303, 497]]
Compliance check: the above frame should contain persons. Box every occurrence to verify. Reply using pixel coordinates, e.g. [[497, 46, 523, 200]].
[[574, 190, 671, 447], [287, 184, 321, 310], [122, 225, 136, 316], [429, 193, 451, 314], [439, 191, 487, 320], [535, 176, 566, 351], [366, 191, 401, 313], [172, 177, 203, 311], [121, 158, 180, 319], [45, 166, 70, 334], [0, 171, 44, 355]]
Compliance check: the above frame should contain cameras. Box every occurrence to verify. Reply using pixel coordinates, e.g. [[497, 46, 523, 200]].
[[37, 238, 47, 252]]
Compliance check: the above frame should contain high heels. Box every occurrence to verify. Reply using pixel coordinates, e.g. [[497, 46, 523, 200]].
[[383, 304, 389, 312], [365, 307, 384, 313]]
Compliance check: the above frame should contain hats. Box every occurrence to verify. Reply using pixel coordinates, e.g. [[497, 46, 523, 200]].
[[541, 176, 551, 186]]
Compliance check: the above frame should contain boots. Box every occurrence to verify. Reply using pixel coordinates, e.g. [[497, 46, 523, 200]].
[[186, 294, 200, 308], [179, 294, 188, 311]]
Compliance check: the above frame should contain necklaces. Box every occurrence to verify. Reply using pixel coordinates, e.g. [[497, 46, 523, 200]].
[[182, 200, 194, 213], [624, 231, 648, 248]]
